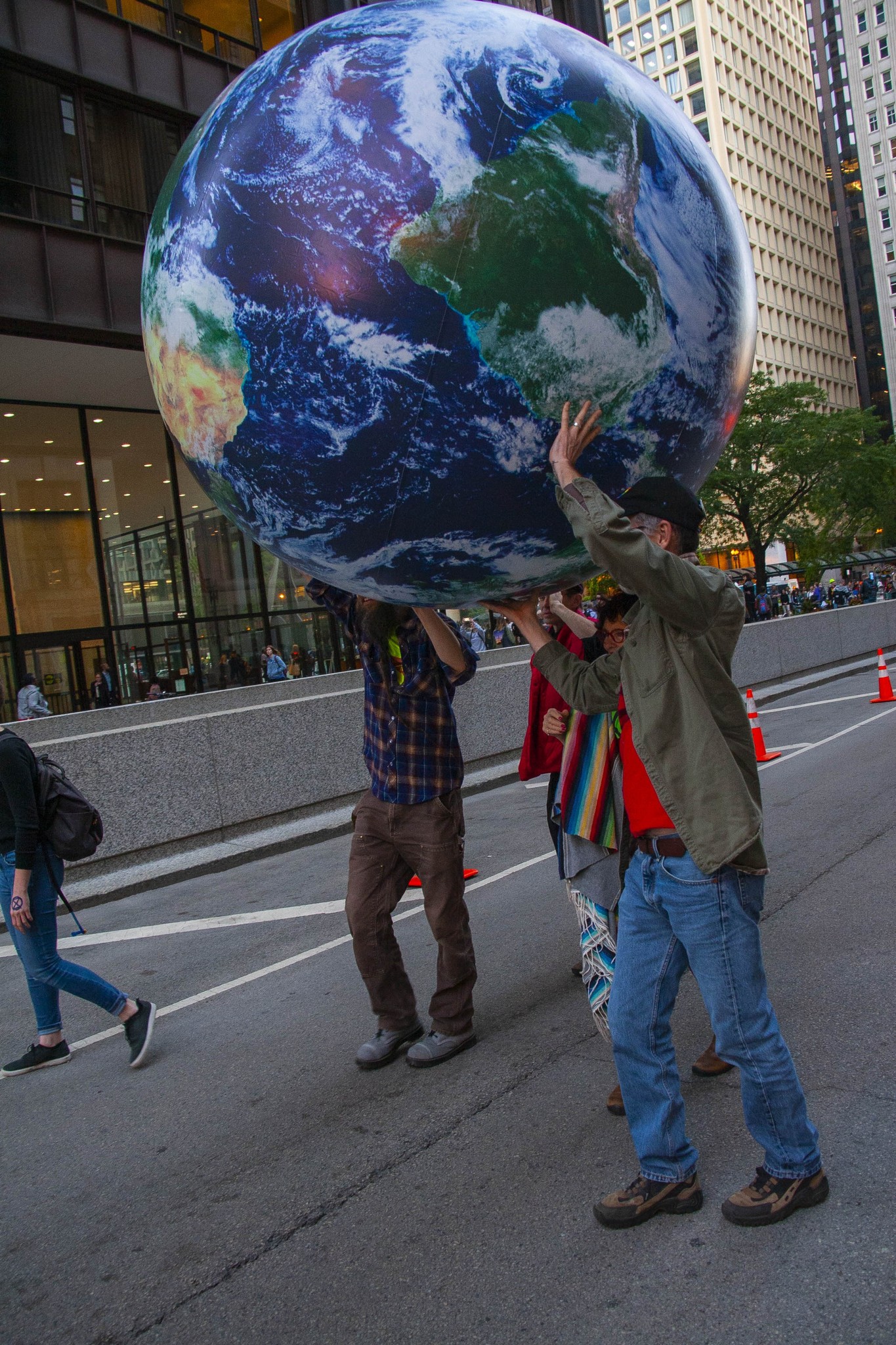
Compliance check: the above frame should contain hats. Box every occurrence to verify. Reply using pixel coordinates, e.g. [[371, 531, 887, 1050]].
[[613, 474, 706, 531]]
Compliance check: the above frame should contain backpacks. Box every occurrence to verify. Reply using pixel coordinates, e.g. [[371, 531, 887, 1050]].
[[728, 573, 896, 621], [0, 730, 103, 938]]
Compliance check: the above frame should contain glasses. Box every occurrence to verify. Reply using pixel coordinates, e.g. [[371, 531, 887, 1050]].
[[596, 628, 626, 643]]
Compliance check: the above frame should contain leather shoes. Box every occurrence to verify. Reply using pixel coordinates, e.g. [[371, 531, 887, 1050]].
[[406, 1027, 477, 1068], [607, 1082, 626, 1116], [690, 1032, 736, 1078], [354, 1013, 426, 1070]]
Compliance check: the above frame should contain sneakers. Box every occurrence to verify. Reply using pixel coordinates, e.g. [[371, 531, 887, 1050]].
[[720, 1161, 828, 1227], [1, 1038, 73, 1077], [593, 1163, 705, 1229], [121, 997, 157, 1068]]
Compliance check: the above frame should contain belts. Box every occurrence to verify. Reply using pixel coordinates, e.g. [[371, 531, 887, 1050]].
[[635, 836, 690, 856]]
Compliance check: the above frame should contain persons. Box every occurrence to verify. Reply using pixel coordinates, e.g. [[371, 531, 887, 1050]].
[[82, 567, 895, 713], [479, 396, 836, 1231], [543, 594, 748, 1115], [16, 672, 54, 721], [515, 585, 607, 975], [302, 577, 482, 1068], [0, 725, 156, 1078]]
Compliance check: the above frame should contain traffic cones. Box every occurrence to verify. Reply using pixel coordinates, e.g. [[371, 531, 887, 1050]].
[[868, 648, 896, 702], [746, 688, 781, 761]]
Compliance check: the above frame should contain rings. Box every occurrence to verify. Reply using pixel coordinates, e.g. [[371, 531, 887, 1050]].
[[570, 421, 582, 429]]
